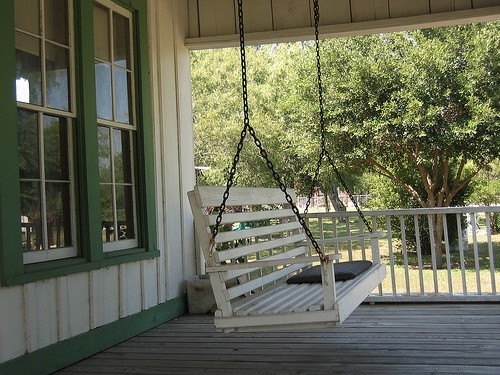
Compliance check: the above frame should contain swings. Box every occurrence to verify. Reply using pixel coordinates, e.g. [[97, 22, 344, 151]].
[[186, 0, 387, 333]]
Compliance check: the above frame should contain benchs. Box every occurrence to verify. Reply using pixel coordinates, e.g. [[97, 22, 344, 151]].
[[186, 183, 388, 333]]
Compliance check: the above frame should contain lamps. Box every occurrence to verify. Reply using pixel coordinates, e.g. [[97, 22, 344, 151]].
[[16, 51, 30, 105]]
